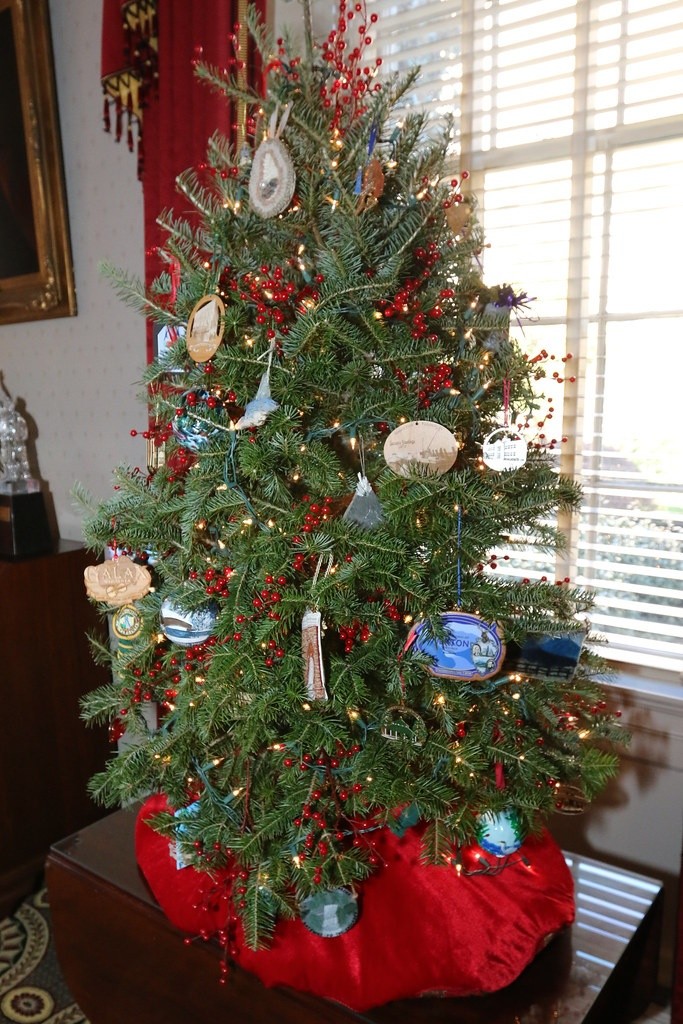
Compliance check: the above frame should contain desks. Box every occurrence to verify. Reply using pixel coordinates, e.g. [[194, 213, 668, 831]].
[[48, 793, 663, 1023]]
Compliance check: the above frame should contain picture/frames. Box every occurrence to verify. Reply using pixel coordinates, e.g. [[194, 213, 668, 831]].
[[1, 0, 78, 327]]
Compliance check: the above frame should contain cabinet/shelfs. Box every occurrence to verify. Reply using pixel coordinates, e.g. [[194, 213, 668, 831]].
[[0, 540, 122, 919]]
